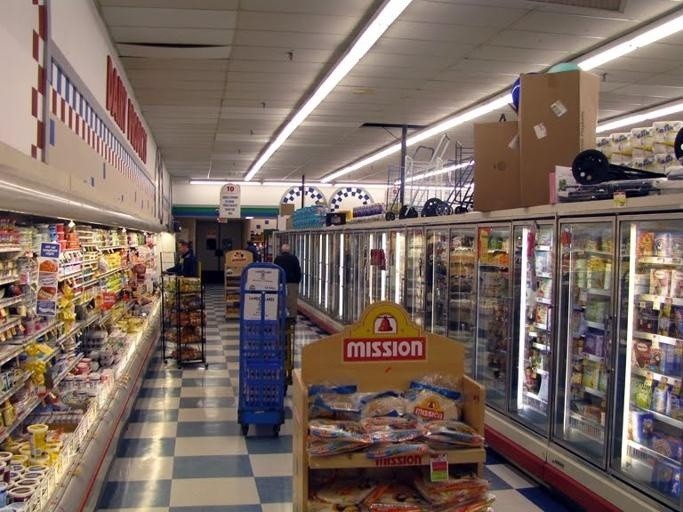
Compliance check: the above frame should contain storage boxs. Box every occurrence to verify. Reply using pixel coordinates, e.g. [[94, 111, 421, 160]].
[[470, 122, 519, 212], [516, 70, 603, 208]]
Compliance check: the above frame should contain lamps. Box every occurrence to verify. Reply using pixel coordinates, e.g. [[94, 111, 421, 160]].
[[317, 2, 682, 182], [391, 97, 682, 189], [241, 1, 413, 183]]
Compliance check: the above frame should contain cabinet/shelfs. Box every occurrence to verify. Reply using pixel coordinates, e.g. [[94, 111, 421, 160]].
[[289, 302, 497, 512], [157, 251, 208, 368], [221, 249, 253, 321]]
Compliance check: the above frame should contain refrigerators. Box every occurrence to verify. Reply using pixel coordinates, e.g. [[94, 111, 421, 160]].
[[511, 204, 559, 447], [284, 211, 515, 434], [614, 194, 683, 510], [553, 197, 619, 473]]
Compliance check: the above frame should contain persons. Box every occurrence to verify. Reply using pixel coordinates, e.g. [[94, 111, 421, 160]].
[[274, 244, 301, 324], [245, 241, 258, 262], [163, 239, 196, 277]]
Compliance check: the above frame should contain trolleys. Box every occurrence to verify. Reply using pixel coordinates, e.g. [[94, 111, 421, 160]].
[[387, 112, 507, 220], [237, 262, 290, 440], [561, 125, 683, 200]]
